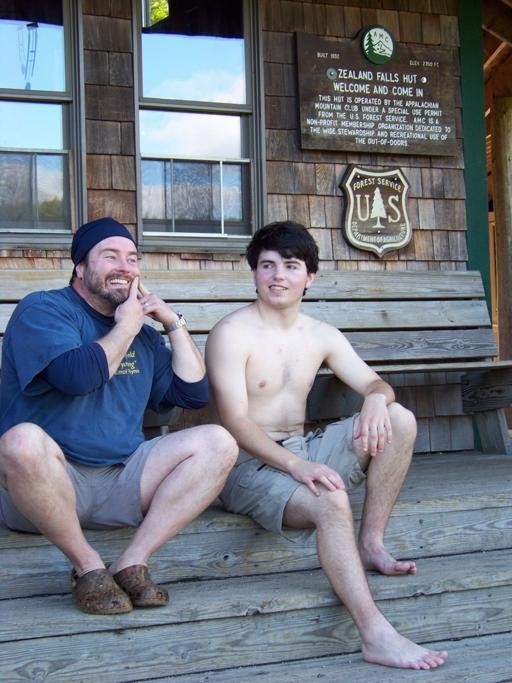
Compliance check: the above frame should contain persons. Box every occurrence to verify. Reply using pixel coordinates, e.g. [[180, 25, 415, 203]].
[[204, 218, 449, 671], [0, 215, 242, 615]]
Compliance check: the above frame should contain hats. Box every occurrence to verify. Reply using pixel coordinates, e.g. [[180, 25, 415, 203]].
[[69, 218, 136, 266]]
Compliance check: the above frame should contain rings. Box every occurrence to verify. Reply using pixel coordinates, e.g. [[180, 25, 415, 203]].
[[145, 301, 150, 307]]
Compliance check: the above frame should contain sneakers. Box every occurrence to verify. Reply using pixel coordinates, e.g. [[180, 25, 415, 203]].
[[107, 560, 168, 606], [70, 569, 133, 613]]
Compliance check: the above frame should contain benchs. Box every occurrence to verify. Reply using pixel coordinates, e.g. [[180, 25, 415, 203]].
[[0, 263, 512, 456]]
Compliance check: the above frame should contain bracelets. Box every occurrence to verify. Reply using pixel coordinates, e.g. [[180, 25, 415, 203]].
[[165, 311, 188, 332]]
